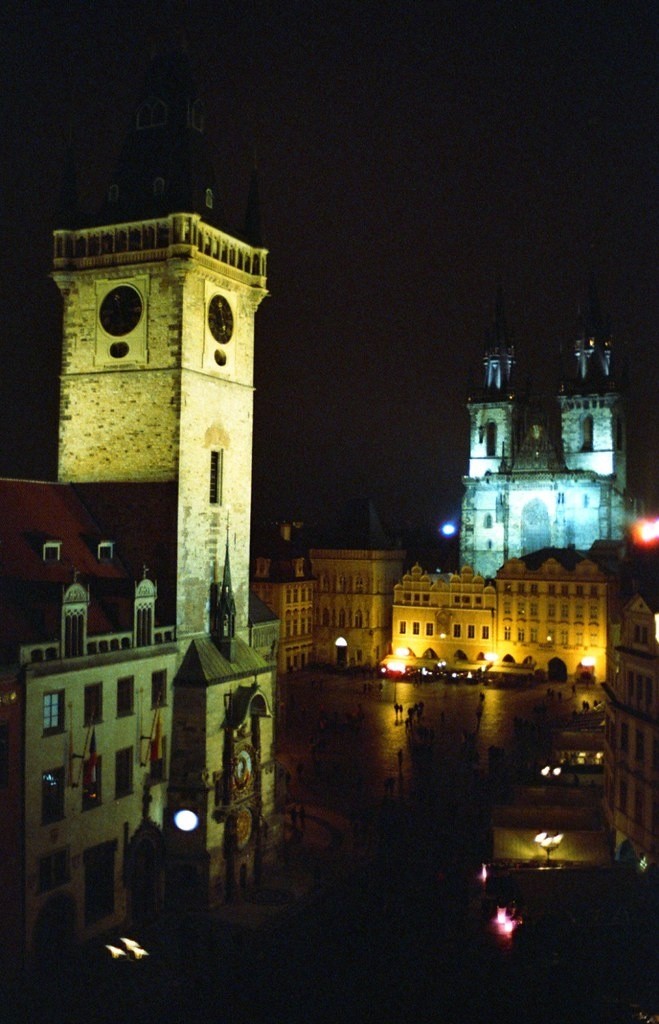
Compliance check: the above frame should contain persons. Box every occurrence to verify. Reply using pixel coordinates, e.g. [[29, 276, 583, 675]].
[[572, 684, 577, 697], [332, 709, 338, 725], [572, 709, 577, 717], [557, 690, 562, 703], [394, 703, 400, 717], [399, 705, 403, 715], [475, 711, 482, 728], [440, 711, 445, 724], [357, 704, 365, 720], [478, 691, 485, 706], [317, 705, 329, 729], [397, 749, 404, 767], [398, 773, 404, 793], [310, 678, 315, 687], [317, 677, 323, 691], [383, 778, 390, 793], [278, 658, 603, 824], [378, 683, 383, 692]]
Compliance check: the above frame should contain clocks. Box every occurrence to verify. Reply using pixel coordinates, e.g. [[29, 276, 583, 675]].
[[99, 286, 142, 337], [208, 295, 233, 344]]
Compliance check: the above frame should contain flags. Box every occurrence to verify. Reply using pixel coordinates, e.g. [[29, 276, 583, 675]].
[[83, 730, 98, 785], [151, 716, 161, 762]]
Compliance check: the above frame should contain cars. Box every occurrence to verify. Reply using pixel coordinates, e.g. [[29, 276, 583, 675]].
[[477, 861, 659, 960]]
